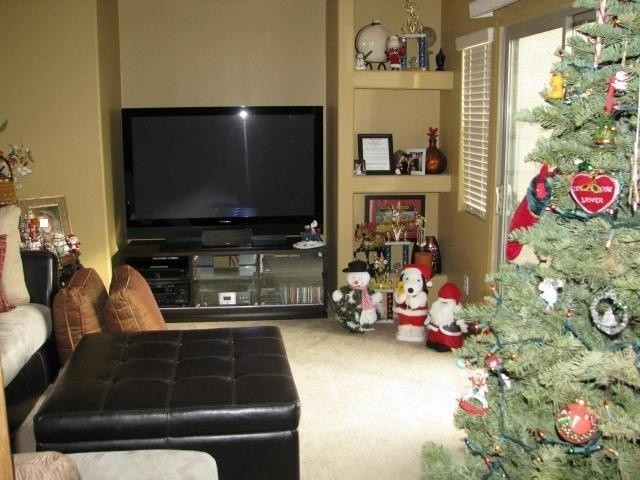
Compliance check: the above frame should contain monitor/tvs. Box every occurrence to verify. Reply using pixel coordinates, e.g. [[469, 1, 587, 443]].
[[119, 105, 326, 245]]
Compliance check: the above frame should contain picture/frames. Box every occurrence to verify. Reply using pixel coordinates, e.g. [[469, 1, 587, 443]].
[[357, 133, 395, 174], [406, 148, 425, 176], [17, 191, 73, 262], [364, 193, 426, 243]]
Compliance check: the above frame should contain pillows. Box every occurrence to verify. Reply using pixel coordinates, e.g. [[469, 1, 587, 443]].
[[101, 264, 167, 331], [53, 267, 110, 367]]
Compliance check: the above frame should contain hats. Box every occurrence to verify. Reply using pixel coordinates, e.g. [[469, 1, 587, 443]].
[[438, 284, 462, 306], [401, 265, 434, 288], [67, 234, 76, 240], [341, 261, 368, 273]]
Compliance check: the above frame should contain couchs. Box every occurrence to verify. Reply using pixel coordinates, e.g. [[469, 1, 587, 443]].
[[33, 326, 302, 480], [12, 450, 220, 480], [0, 201, 58, 435]]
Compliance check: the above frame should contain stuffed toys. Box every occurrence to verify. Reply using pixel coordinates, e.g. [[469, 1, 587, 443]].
[[301, 220, 321, 240], [387, 34, 407, 70], [393, 263, 432, 342], [332, 260, 382, 334], [424, 282, 468, 352], [66, 233, 80, 252]]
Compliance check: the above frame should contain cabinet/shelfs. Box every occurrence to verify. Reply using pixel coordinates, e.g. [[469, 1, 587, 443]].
[[110, 237, 331, 321]]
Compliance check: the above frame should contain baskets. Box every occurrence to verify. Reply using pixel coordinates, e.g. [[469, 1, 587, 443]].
[[0, 155, 18, 206]]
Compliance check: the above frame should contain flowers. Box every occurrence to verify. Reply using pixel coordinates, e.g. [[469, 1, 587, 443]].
[[0, 118, 37, 184]]
